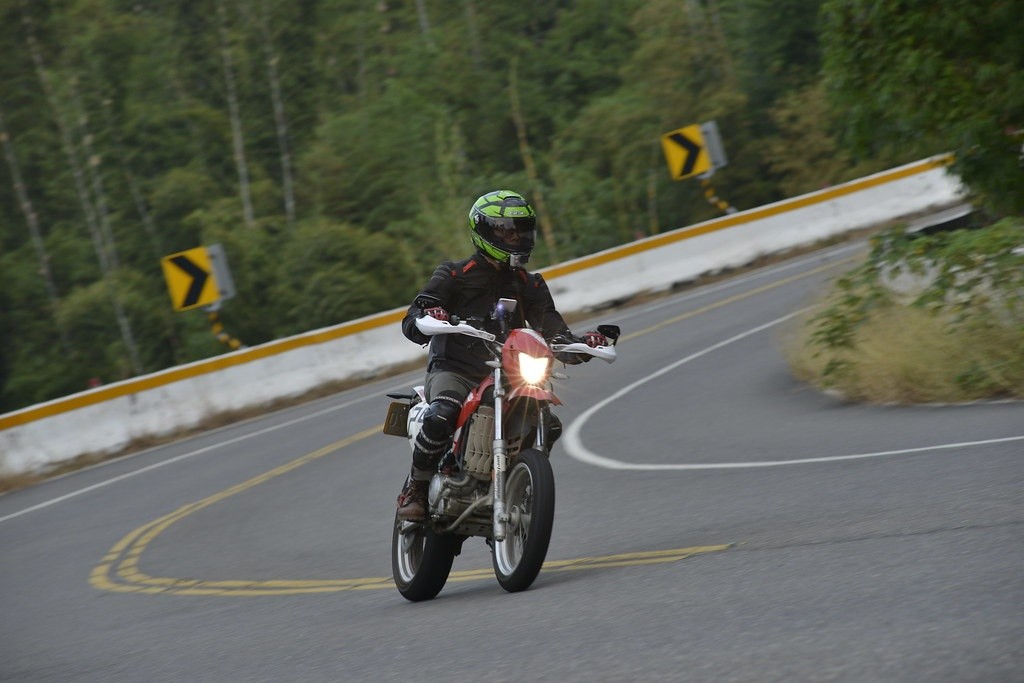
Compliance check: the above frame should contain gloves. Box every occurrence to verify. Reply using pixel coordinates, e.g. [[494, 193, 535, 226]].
[[575, 330, 608, 363], [420, 305, 450, 323]]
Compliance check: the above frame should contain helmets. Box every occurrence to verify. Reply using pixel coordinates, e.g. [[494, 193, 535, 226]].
[[467, 189, 536, 272]]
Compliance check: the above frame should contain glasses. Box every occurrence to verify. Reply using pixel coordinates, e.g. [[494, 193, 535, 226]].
[[496, 229, 521, 239]]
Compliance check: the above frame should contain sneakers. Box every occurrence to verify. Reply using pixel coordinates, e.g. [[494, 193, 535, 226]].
[[397, 485, 428, 522]]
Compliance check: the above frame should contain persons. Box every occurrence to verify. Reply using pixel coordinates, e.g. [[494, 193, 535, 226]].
[[398, 190, 607, 521]]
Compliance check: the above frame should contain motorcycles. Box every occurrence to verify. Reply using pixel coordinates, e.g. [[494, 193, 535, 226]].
[[381, 297, 621, 603]]
